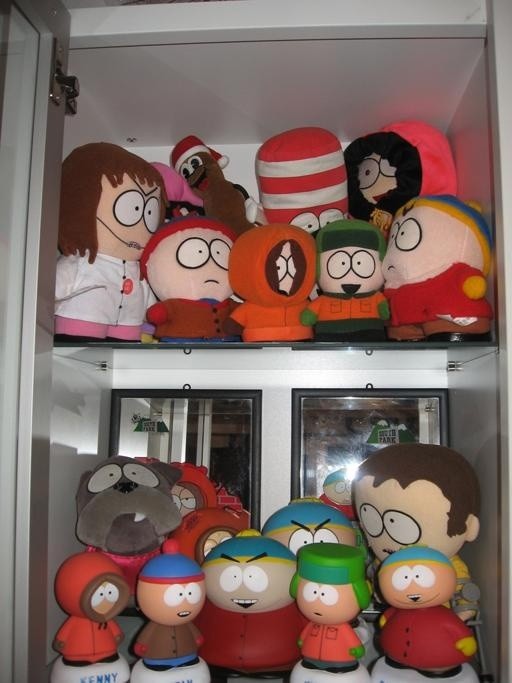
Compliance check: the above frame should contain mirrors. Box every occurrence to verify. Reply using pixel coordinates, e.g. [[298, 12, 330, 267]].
[[290, 387, 451, 504], [108, 387, 263, 533]]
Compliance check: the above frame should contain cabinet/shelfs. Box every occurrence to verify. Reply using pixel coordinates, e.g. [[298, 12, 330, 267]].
[[16, 0, 512, 683]]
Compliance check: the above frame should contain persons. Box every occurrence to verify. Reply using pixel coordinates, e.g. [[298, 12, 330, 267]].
[[376, 542, 481, 679], [188, 529, 310, 678], [124, 551, 208, 672], [261, 496, 359, 557], [319, 466, 360, 522], [52, 549, 131, 668], [52, 495, 481, 679], [74, 454, 184, 613], [194, 525, 243, 566], [289, 541, 372, 673], [352, 443, 480, 639], [56, 122, 493, 342], [166, 461, 217, 521]]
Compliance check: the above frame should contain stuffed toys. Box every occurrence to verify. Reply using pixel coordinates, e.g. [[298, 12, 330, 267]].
[[56, 122, 493, 342]]
